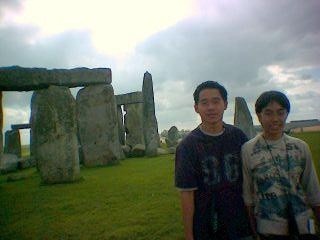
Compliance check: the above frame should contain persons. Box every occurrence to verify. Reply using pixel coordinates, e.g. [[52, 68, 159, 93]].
[[174, 81, 252, 240], [241, 91, 320, 240]]
